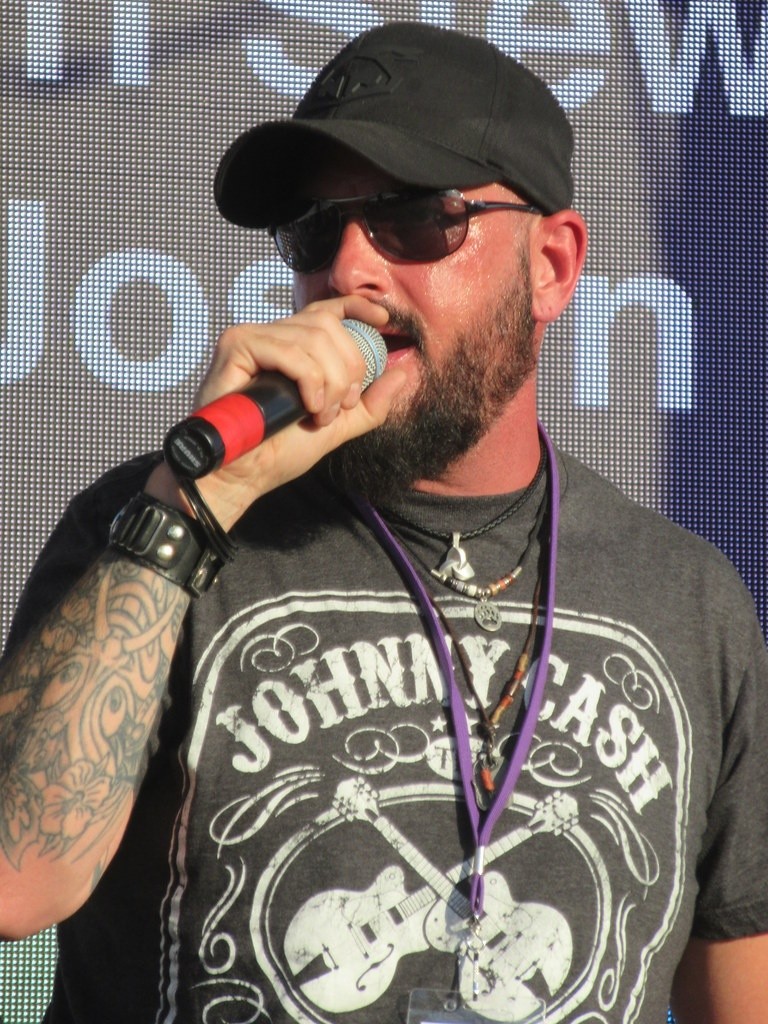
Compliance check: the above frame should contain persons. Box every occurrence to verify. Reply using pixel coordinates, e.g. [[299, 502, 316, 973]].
[[2, 20, 768, 1024]]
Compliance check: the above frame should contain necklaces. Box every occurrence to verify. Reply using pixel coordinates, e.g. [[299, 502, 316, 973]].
[[377, 434, 555, 812]]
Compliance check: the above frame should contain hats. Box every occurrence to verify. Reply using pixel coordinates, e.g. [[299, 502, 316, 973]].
[[213, 22, 575, 229]]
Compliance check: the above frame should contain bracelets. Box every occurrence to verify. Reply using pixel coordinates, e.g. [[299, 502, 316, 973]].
[[110, 493, 224, 599]]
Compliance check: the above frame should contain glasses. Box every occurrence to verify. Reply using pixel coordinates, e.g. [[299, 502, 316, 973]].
[[267, 181, 540, 274]]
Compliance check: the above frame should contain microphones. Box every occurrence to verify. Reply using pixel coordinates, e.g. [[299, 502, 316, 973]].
[[162, 319, 387, 481]]
